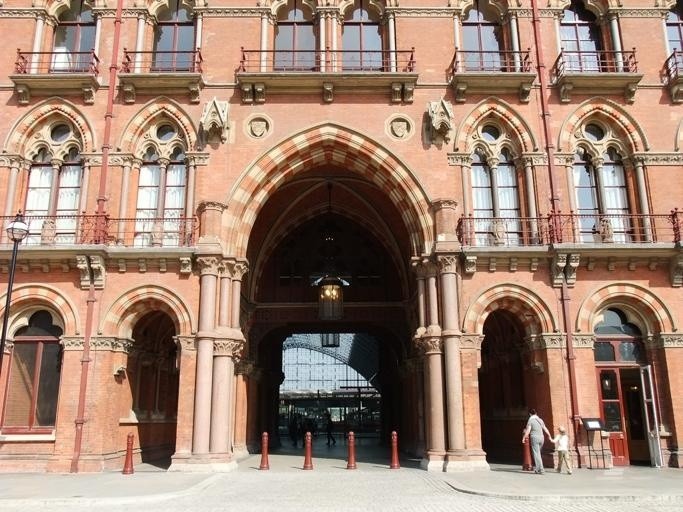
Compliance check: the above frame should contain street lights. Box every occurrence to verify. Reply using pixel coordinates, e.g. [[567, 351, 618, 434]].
[[0, 209, 28, 374]]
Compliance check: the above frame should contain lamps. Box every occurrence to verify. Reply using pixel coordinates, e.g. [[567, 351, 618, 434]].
[[308, 184, 350, 347]]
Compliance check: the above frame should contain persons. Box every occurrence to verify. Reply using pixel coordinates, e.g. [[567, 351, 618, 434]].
[[524, 407, 555, 476], [554, 425, 574, 476], [51, 41, 75, 72], [288, 405, 359, 448]]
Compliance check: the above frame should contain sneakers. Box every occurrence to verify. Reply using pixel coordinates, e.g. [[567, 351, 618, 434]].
[[535, 470, 544, 475], [555, 468, 561, 472], [568, 471, 572, 474]]
